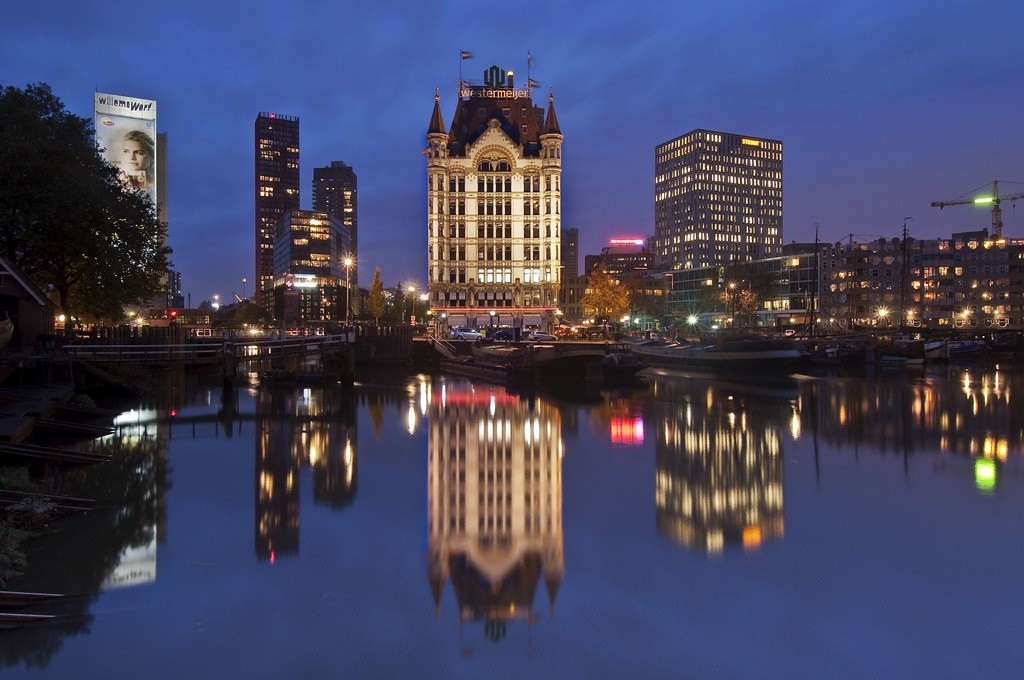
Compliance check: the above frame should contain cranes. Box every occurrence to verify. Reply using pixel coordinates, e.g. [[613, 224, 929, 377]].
[[931, 178, 1024, 236]]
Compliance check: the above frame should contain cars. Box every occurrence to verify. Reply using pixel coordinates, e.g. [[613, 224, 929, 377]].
[[531, 330, 558, 342], [455, 328, 486, 340], [495, 330, 523, 340], [647, 332, 667, 343], [784, 329, 795, 337]]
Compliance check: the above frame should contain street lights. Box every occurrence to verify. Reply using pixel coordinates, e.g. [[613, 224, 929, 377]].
[[341, 253, 354, 327], [243, 278, 247, 301], [663, 274, 675, 318]]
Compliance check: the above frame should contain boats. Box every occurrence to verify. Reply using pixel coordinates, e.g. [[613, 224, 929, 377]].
[[472, 340, 554, 370], [951, 342, 981, 353], [259, 369, 340, 384], [925, 338, 950, 360], [0, 311, 14, 348], [812, 350, 908, 367], [628, 336, 810, 373]]
